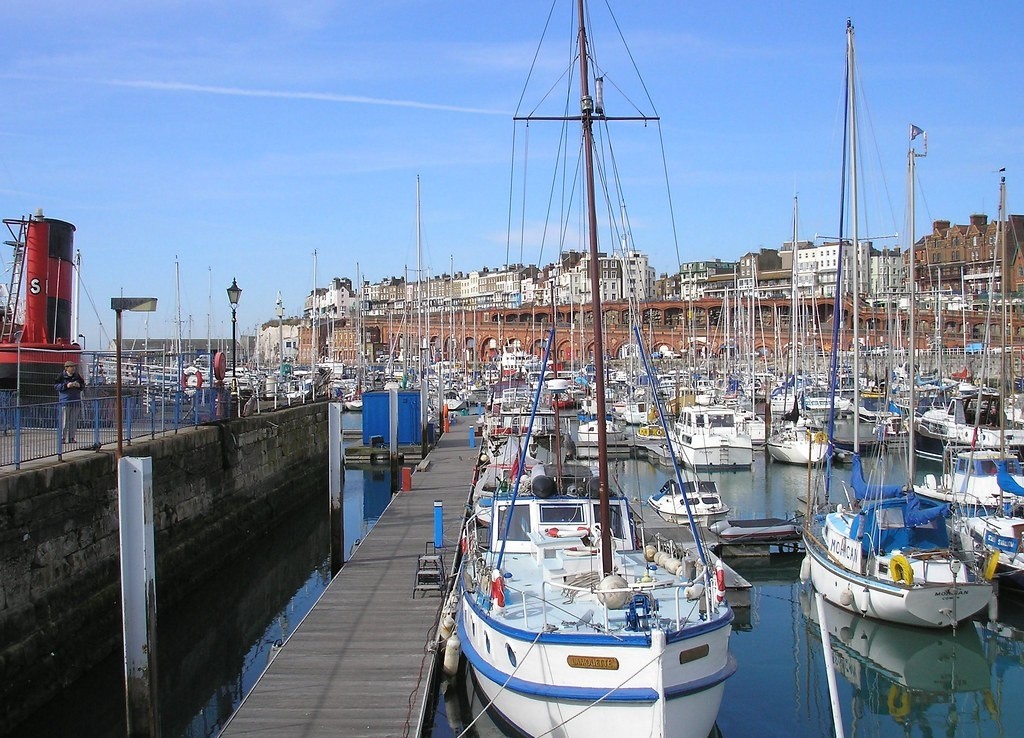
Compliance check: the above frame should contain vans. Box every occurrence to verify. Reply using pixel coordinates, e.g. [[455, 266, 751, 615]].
[[196, 354, 210, 362]]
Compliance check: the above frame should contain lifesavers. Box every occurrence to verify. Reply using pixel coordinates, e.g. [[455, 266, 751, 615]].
[[984, 549, 1000, 581], [887, 683, 911, 718], [461, 534, 466, 554], [982, 689, 998, 713], [890, 556, 914, 586], [815, 431, 827, 444], [548, 525, 590, 538], [181, 366, 203, 392], [715, 558, 726, 604], [490, 568, 505, 609], [640, 427, 650, 435], [563, 546, 600, 557], [654, 427, 665, 437]]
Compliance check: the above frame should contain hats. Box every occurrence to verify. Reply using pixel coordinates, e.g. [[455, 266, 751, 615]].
[[64, 361, 78, 367]]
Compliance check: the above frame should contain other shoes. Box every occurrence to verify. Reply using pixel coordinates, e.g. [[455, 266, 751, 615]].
[[62, 440, 66, 444], [69, 438, 77, 444]]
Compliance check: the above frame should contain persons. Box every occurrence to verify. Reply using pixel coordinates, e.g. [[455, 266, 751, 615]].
[[989, 408, 1000, 428], [53, 361, 86, 444]]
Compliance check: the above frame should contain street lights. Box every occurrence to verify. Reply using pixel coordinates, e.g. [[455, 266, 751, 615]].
[[111, 297, 157, 461], [227, 277, 242, 397]]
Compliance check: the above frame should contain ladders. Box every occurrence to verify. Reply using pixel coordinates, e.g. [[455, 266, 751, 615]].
[[0, 213, 33, 342]]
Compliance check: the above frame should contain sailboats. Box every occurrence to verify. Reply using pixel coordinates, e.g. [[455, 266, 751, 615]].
[[93, 18, 1024, 628], [453, 0, 734, 738], [801, 583, 1001, 738]]
